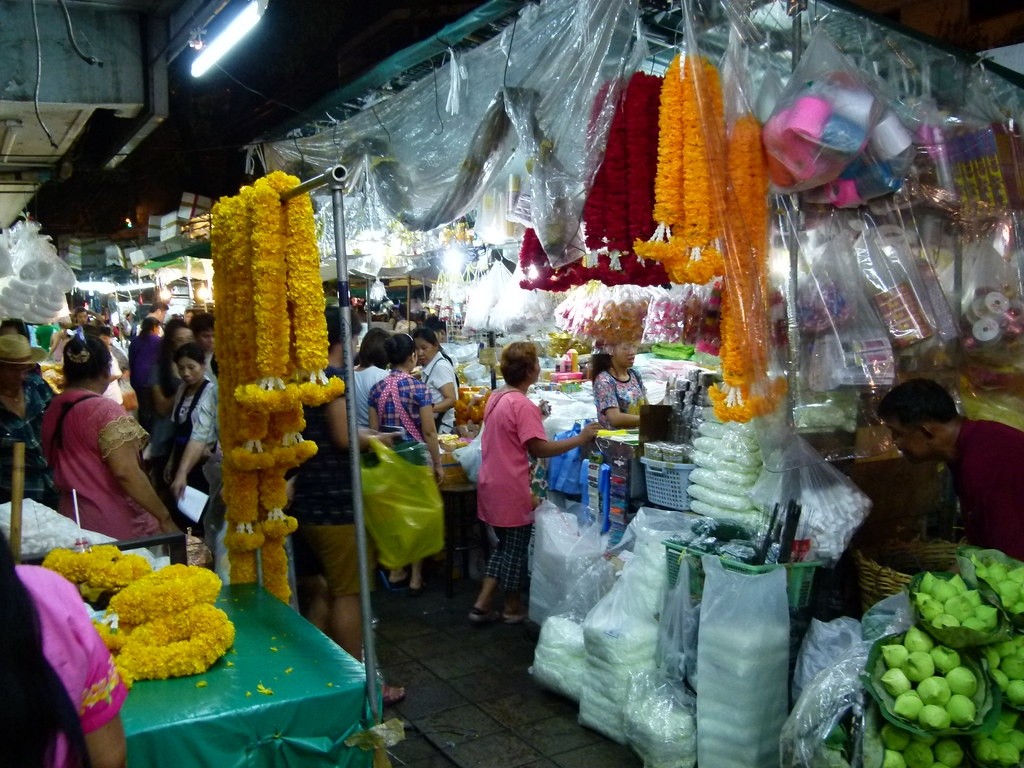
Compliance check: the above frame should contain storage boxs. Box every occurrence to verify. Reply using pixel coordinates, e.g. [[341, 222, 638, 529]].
[[639, 458, 694, 510], [660, 538, 817, 610]]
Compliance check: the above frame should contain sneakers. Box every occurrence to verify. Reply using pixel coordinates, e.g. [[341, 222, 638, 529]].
[[502, 605, 530, 623], [466, 603, 498, 623]]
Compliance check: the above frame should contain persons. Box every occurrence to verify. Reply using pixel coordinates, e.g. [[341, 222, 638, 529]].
[[0, 298, 459, 768], [470, 343, 603, 619], [880, 378, 1024, 561], [593, 340, 651, 430]]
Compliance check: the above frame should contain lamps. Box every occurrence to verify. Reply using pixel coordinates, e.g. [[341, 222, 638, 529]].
[[190, 0, 271, 78]]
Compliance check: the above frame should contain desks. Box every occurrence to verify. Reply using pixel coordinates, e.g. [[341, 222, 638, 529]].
[[91, 579, 372, 767], [438, 484, 477, 599]]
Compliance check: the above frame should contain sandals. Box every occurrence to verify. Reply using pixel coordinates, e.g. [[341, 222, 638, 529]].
[[381, 685, 408, 709]]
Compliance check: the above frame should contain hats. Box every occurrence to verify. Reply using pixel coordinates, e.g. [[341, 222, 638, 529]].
[[1, 334, 48, 366]]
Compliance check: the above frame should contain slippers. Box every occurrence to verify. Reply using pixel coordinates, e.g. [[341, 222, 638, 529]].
[[388, 572, 412, 588], [405, 575, 427, 598]]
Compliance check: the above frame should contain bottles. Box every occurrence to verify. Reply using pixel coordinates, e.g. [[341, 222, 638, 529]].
[[73, 537, 90, 553], [565, 357, 571, 372], [554, 354, 562, 372]]
